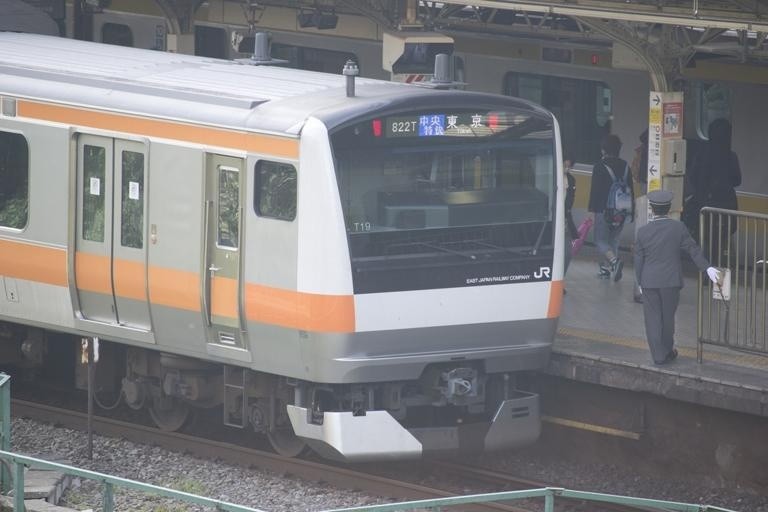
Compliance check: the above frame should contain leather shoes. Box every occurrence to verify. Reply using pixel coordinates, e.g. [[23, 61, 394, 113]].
[[666, 349, 678, 361]]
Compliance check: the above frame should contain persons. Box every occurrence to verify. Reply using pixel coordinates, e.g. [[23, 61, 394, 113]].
[[684, 118, 742, 285], [564, 155, 579, 294], [634, 189, 721, 363], [585, 135, 639, 280], [630, 126, 648, 194]]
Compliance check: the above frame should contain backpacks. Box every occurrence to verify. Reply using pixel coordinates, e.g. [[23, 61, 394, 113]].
[[603, 163, 634, 231]]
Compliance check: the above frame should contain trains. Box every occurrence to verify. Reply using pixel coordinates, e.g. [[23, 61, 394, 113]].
[[0, 29, 574, 464]]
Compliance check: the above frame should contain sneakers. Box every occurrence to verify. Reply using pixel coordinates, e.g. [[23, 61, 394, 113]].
[[597, 270, 609, 280], [609, 258, 624, 282]]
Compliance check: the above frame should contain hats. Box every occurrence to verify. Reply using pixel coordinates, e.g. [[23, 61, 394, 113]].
[[647, 190, 674, 208]]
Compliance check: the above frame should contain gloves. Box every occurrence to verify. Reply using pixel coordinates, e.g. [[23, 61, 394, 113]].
[[706, 267, 721, 284]]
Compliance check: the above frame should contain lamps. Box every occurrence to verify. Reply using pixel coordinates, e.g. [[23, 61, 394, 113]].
[[296, 0, 338, 30], [239, 0, 266, 37]]
